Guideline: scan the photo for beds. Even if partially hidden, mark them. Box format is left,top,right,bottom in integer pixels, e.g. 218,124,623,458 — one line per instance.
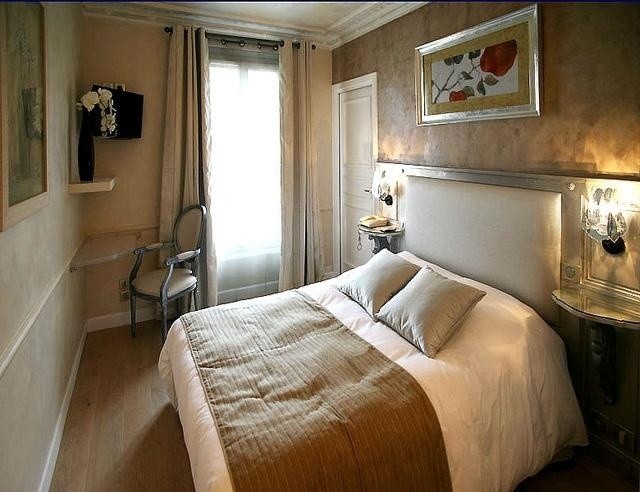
154,179,587,491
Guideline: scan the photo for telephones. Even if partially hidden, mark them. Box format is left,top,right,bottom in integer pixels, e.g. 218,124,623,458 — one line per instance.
359,215,389,227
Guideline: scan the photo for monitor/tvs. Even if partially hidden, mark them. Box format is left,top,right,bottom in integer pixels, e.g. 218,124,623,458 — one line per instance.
90,84,144,140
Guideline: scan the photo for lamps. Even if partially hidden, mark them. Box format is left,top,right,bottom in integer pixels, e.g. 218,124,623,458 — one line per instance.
371,179,393,206
581,205,628,254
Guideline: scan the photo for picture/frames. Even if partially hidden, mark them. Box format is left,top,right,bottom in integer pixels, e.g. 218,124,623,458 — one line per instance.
1,1,53,232
412,3,543,128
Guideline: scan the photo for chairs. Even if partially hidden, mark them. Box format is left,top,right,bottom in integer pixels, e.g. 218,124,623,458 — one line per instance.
128,203,208,347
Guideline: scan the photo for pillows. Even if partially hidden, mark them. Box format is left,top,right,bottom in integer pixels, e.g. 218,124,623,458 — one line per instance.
333,247,488,361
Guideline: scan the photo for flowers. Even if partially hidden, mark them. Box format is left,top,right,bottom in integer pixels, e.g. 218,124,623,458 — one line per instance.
76,88,119,139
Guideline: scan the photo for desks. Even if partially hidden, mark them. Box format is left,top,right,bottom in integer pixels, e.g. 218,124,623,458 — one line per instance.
551,289,640,406
358,225,403,258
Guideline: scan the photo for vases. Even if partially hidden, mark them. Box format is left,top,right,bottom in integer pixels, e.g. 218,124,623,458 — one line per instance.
77,105,95,182
